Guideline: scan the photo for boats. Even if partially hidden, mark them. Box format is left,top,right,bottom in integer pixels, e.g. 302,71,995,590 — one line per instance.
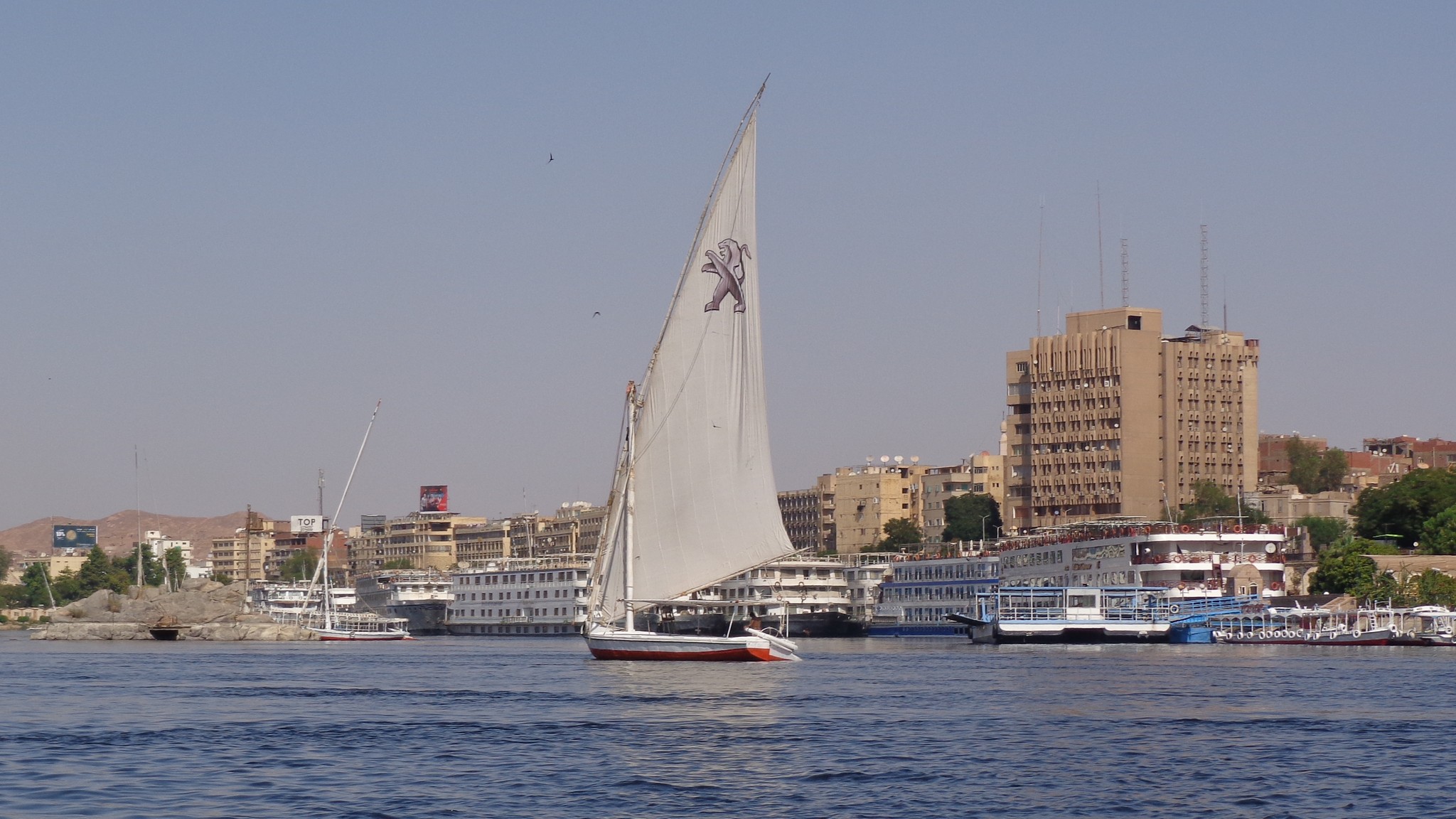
149,626,192,640
653,612,727,637
1301,623,1397,645
1168,613,1218,644
735,611,853,638
940,585,1174,647
1418,623,1456,646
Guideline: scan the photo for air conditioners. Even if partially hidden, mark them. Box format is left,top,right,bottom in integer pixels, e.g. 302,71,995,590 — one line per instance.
1062,448,1067,453
1075,469,1080,474
1193,427,1199,432
1035,490,1115,498
1188,427,1192,431
1091,446,1098,451
1031,381,1110,394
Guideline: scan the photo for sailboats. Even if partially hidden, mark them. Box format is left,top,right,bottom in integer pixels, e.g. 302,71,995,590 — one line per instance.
297,400,413,641
581,70,804,664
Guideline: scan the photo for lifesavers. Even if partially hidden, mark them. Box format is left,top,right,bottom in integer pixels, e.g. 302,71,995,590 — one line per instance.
777,595,782,601
356,569,448,583
1169,604,1180,614
1445,625,1453,634
1215,625,1362,641
775,581,780,587
799,582,804,587
1390,624,1397,633
1281,554,1286,562
893,549,989,562
1234,525,1242,533
1271,582,1279,590
1004,526,1151,551
1181,525,1189,533
1173,555,1181,563
1177,582,1187,589
1154,555,1161,564
801,595,806,601
1249,554,1256,563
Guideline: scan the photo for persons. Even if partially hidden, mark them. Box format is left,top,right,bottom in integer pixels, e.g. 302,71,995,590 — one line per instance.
420,493,442,511
743,612,761,636
